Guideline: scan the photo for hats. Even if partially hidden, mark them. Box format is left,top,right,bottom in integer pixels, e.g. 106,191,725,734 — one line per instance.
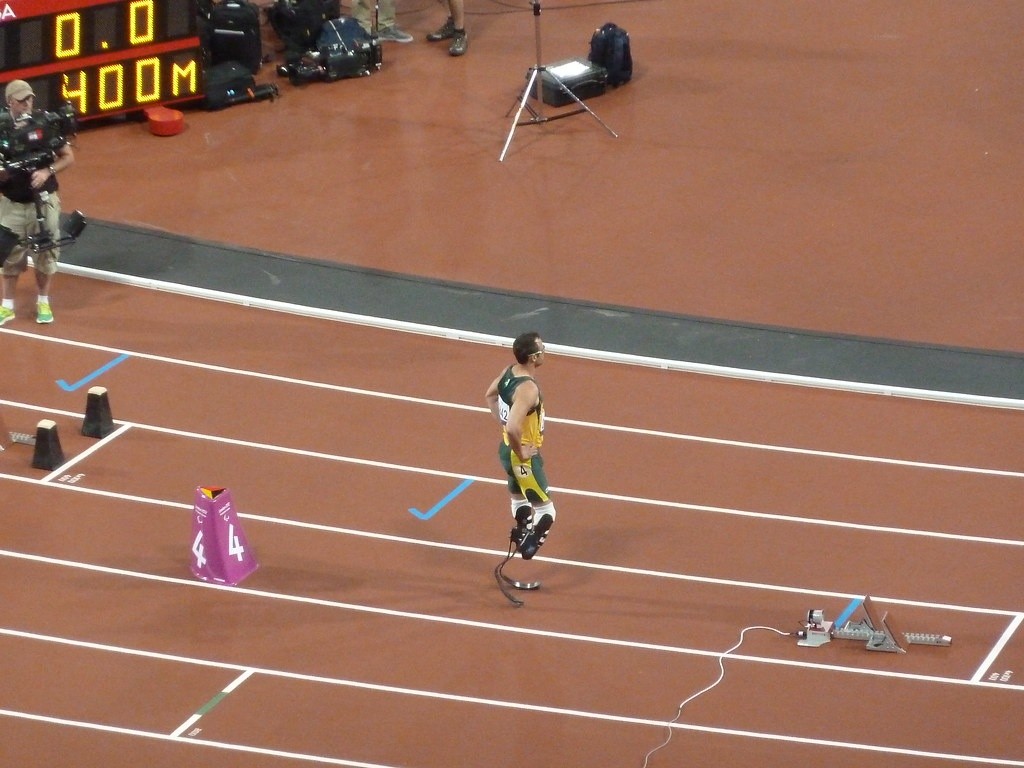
5,79,36,100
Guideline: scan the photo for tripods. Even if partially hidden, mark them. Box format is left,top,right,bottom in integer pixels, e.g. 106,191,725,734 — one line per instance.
498,0,618,162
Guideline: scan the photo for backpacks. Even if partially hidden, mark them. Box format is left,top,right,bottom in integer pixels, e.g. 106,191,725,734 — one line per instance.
317,16,370,52
588,23,632,88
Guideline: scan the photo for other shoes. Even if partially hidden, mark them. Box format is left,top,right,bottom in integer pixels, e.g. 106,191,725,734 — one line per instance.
378,26,413,43
449,33,468,56
426,23,454,41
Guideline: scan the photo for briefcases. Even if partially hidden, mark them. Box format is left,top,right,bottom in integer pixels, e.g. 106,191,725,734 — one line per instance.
209,0,261,77
525,57,608,108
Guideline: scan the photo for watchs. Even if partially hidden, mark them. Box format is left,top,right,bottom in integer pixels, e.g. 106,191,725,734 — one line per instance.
47,164,56,175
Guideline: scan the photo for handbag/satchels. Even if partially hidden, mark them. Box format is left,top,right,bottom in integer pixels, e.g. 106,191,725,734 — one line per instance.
265,0,341,48
205,61,255,105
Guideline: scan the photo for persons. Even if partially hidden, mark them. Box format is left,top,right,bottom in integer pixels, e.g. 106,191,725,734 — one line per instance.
351,0,414,49
426,0,469,56
0,78,76,326
485,331,558,561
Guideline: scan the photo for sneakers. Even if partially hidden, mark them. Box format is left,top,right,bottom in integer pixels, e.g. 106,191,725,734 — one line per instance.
0,306,15,325
36,302,53,323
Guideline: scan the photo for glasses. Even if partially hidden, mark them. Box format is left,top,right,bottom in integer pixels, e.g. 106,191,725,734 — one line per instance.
528,346,545,357
